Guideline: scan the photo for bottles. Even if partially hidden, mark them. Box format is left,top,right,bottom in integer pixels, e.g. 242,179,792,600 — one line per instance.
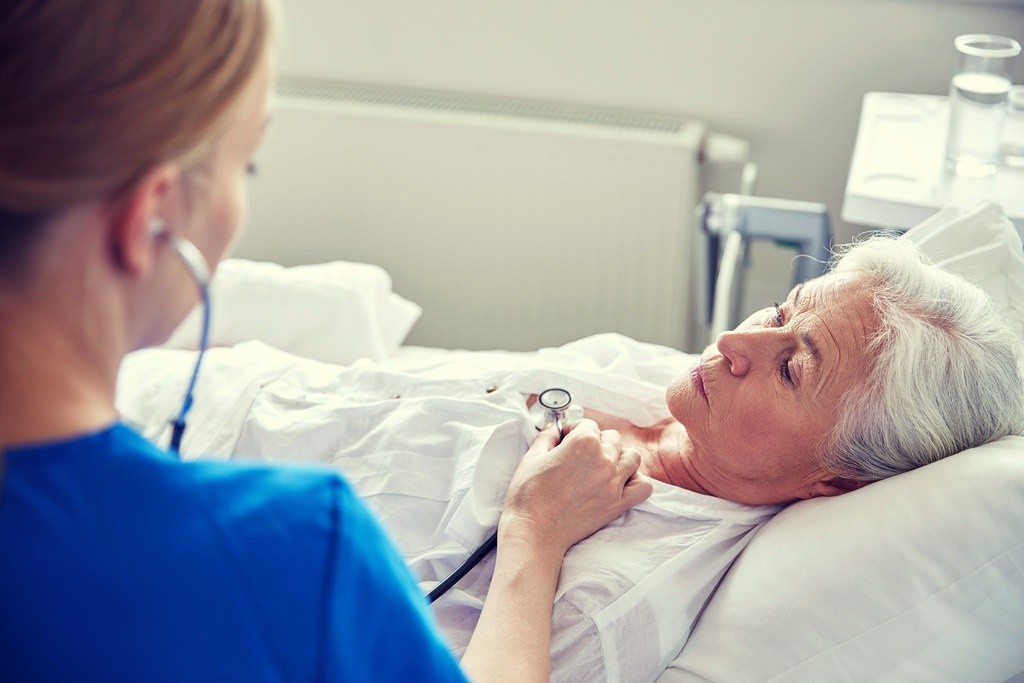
946,74,1010,179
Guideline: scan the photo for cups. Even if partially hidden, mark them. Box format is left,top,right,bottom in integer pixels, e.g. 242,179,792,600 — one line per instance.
955,33,1022,81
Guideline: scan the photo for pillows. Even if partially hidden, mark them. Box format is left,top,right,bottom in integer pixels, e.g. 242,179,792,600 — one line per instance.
654,204,1024,682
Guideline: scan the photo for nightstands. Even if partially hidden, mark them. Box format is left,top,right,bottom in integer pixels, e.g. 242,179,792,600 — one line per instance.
839,89,1024,238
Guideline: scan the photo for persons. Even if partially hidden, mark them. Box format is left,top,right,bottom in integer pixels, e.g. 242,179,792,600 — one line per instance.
114,233,1024,683
0,0,650,683
